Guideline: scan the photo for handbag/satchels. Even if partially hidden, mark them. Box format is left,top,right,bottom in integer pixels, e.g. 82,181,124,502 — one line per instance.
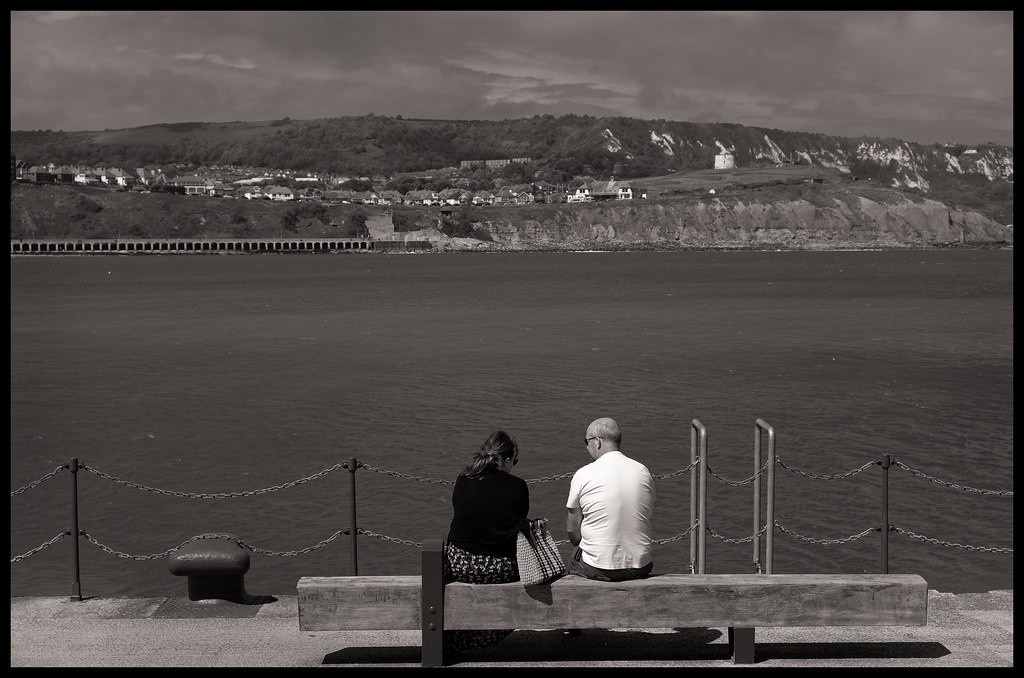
517,516,567,586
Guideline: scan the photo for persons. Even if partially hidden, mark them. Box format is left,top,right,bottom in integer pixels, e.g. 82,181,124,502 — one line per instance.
566,418,657,634
446,430,529,653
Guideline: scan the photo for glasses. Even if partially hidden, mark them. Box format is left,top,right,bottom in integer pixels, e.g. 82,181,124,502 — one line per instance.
508,457,519,466
584,436,604,446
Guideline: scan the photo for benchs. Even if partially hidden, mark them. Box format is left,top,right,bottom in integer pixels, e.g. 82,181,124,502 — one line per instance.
297,574,929,663
